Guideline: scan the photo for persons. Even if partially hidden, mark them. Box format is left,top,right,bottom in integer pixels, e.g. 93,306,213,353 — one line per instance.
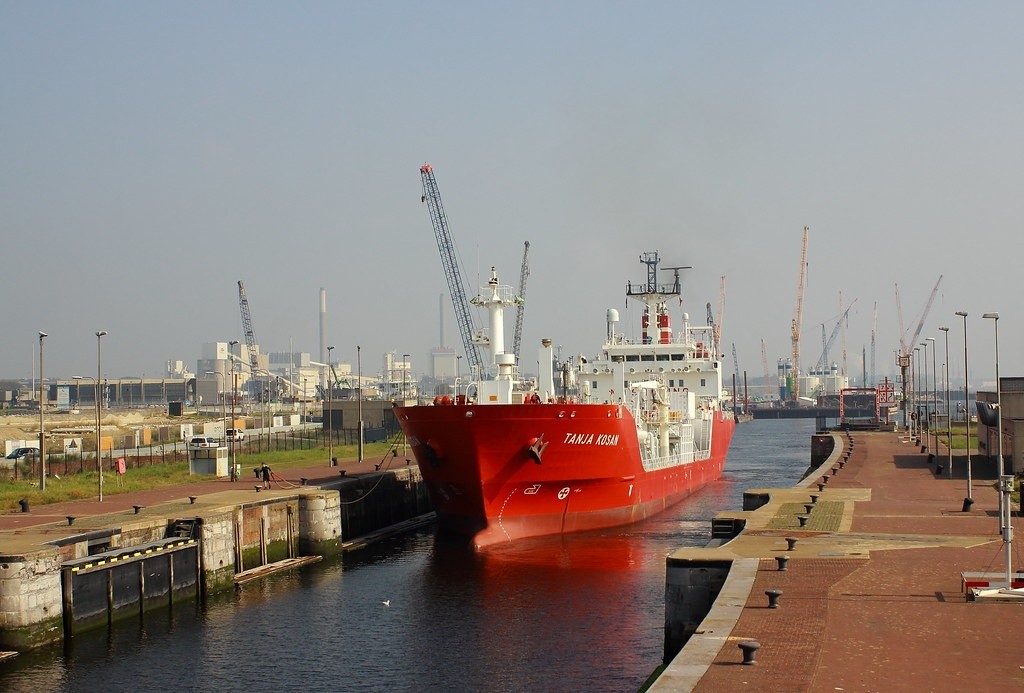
259,462,272,490
390,399,399,408
531,392,543,403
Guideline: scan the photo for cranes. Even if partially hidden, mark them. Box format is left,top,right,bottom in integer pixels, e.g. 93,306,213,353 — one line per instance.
731,343,741,393
511,240,530,366
419,160,486,382
761,338,771,394
713,275,725,353
791,224,809,402
237,280,262,402
816,289,859,378
870,302,877,388
894,273,944,379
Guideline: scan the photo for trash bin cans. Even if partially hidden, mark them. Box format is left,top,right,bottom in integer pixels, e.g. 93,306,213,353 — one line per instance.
1019,481,1024,515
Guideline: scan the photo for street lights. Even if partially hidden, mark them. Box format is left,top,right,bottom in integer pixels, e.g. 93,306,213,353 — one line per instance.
207,340,270,483
909,338,939,466
955,312,972,499
982,312,1004,535
72,331,110,502
939,327,952,478
456,356,463,395
403,354,410,457
357,346,363,463
39,332,46,492
327,346,335,468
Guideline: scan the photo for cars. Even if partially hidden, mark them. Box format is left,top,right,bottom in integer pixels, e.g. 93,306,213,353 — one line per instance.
226,428,244,442
2,447,40,466
190,436,219,448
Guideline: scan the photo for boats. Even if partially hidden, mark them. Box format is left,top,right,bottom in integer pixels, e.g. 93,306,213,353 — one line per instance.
390,250,736,551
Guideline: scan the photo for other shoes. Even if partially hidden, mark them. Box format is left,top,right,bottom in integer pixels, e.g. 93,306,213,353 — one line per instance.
269,485,271,489
264,487,267,490
